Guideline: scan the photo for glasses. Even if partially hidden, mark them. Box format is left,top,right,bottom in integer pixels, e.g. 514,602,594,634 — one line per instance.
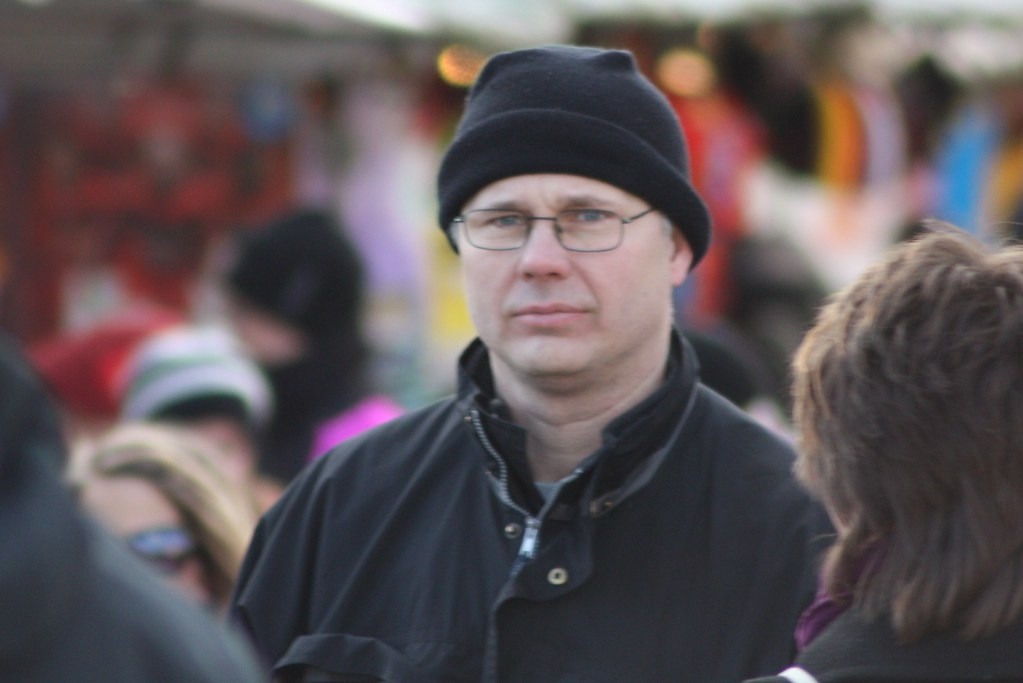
121,527,200,575
449,205,657,253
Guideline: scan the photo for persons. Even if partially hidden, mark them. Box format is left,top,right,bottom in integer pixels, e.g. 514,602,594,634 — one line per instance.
734,216,1023,683
222,206,377,487
223,43,838,683
69,417,255,620
114,319,286,513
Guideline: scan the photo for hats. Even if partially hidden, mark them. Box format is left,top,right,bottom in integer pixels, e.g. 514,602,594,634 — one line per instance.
118,324,274,426
235,212,361,327
437,49,713,274
